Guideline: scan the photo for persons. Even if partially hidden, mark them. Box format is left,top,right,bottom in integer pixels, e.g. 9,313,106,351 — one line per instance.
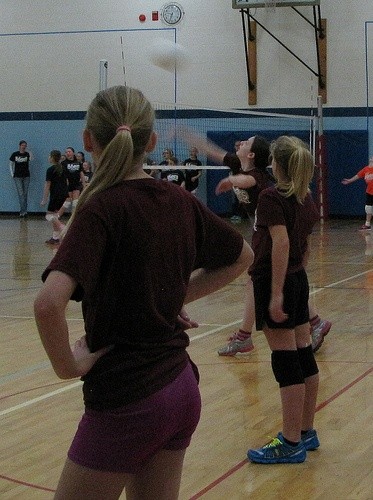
58,145,86,219
167,122,332,357
228,139,244,221
59,151,94,198
34,86,254,500
183,146,202,199
40,149,70,246
9,141,34,221
248,137,320,465
144,148,187,188
342,156,373,232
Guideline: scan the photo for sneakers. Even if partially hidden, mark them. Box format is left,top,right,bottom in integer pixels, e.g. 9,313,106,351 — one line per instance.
302,428,320,451
218,331,254,357
248,432,306,464
310,319,331,351
358,224,371,230
231,215,241,224
45,237,60,245
